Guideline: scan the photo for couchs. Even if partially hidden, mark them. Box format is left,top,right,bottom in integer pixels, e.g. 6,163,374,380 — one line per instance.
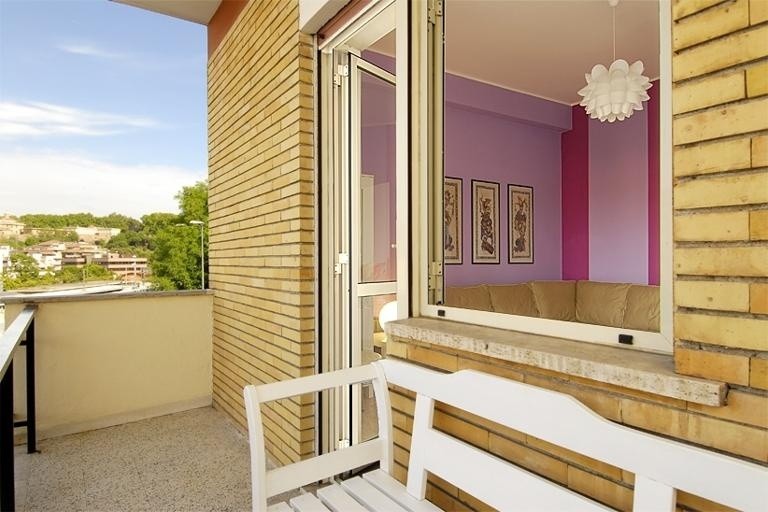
445,279,660,332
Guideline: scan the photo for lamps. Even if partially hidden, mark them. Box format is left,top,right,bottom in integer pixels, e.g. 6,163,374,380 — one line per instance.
577,1,653,123
379,299,398,343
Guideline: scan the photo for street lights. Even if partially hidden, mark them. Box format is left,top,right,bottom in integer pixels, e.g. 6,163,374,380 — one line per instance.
131,255,137,285
189,219,206,290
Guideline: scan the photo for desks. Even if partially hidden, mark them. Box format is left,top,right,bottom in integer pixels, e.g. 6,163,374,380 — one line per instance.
373,330,386,359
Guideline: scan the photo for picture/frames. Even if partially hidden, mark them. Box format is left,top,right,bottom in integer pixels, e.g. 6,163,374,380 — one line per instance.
443,178,463,264
469,178,501,266
506,183,536,263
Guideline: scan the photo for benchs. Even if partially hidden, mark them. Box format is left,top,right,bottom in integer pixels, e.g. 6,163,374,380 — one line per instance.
242,357,768,511
